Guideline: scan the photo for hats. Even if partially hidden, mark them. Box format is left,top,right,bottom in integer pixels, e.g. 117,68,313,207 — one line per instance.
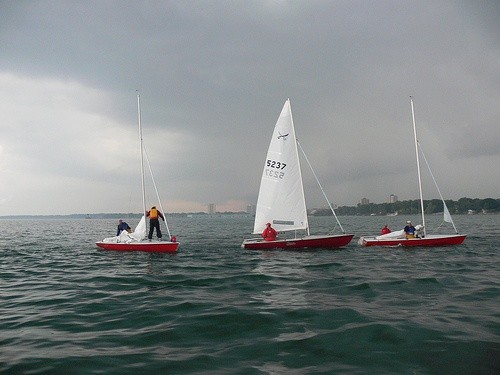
266,223,271,227
407,221,411,223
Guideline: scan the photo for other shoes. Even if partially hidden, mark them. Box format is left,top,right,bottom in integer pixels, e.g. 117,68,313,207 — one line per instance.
159,237,161,239
149,239,151,241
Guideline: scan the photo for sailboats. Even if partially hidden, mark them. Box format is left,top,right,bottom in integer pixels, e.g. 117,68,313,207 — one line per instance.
241,97,355,249
95,94,180,252
358,99,467,247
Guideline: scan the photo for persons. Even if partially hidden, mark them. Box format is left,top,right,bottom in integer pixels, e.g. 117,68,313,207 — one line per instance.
261,223,278,241
404,220,416,235
381,224,391,235
116,219,132,236
146,206,164,239
